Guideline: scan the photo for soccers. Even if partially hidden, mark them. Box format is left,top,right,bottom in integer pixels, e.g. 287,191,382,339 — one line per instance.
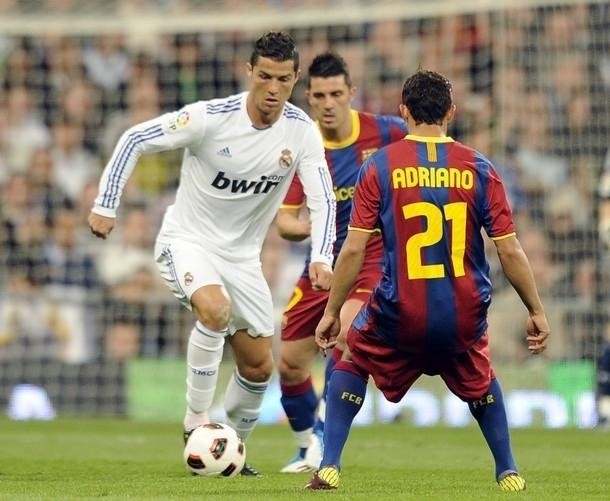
184,423,246,478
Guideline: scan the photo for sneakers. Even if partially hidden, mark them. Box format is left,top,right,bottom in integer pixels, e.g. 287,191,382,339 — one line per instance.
497,469,527,492
183,419,201,444
239,464,259,475
278,426,342,489
